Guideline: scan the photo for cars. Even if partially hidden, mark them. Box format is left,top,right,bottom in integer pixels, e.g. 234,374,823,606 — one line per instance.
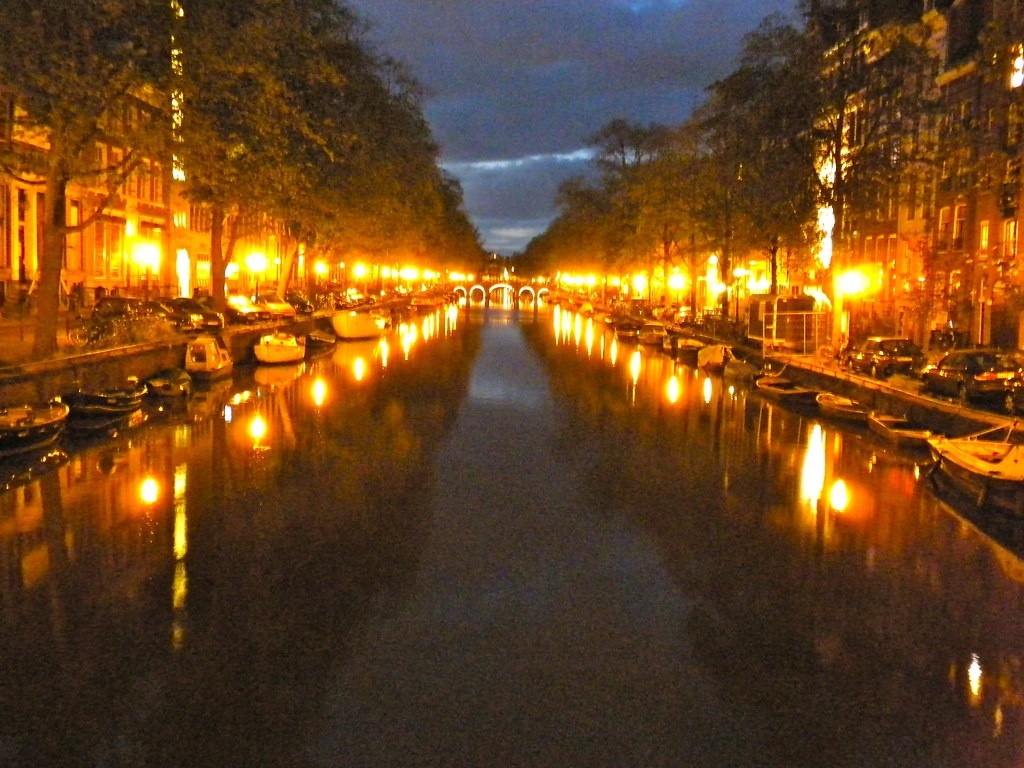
843,335,926,380
920,348,1024,410
88,290,315,344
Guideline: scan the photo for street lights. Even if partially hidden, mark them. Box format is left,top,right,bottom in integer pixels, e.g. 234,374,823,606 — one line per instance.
250,253,266,301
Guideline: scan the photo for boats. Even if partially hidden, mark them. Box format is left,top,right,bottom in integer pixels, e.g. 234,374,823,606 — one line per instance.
142,366,191,398
70,380,148,417
331,313,384,339
865,410,931,448
370,307,394,326
309,329,336,345
406,291,462,308
185,335,234,381
756,375,819,407
815,392,869,424
0,401,70,457
592,311,735,371
254,329,307,364
925,437,1024,519
